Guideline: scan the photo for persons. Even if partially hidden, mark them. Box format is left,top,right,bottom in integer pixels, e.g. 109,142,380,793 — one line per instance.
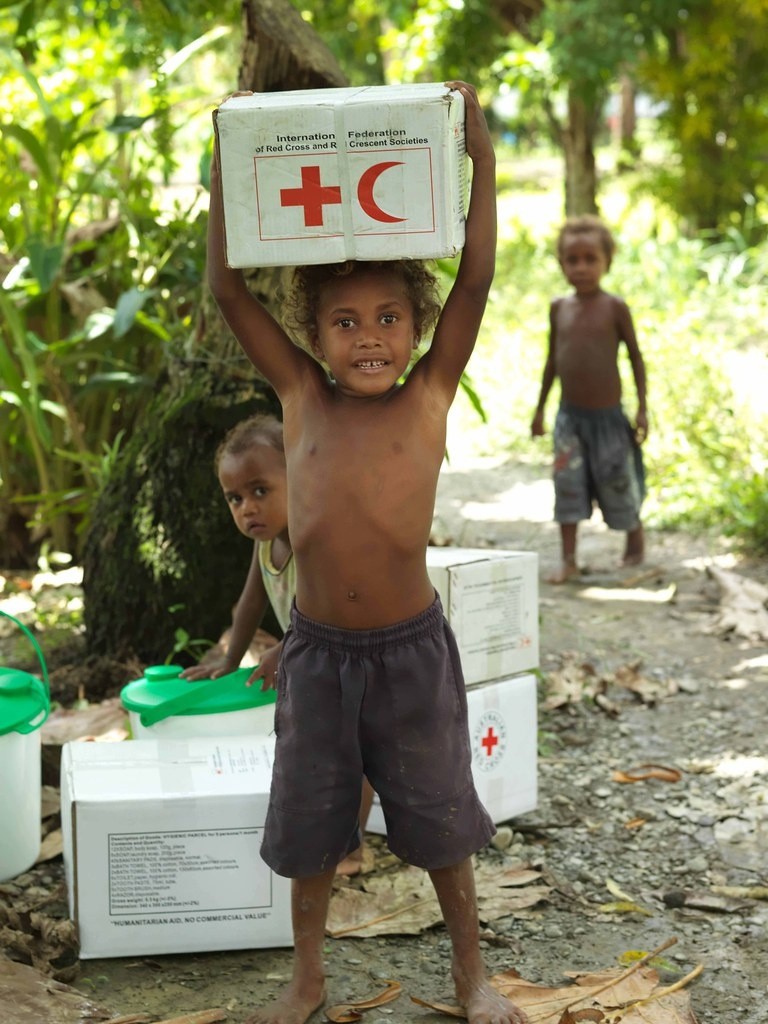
208,82,532,1024
533,216,648,585
179,416,373,877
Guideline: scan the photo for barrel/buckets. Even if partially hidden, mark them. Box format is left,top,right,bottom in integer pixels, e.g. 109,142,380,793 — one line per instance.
118,665,279,739
0,609,50,882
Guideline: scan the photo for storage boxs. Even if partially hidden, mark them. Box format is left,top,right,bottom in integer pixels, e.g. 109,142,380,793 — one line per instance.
364,672,539,835
60,738,294,959
214,79,466,270
425,545,540,684
